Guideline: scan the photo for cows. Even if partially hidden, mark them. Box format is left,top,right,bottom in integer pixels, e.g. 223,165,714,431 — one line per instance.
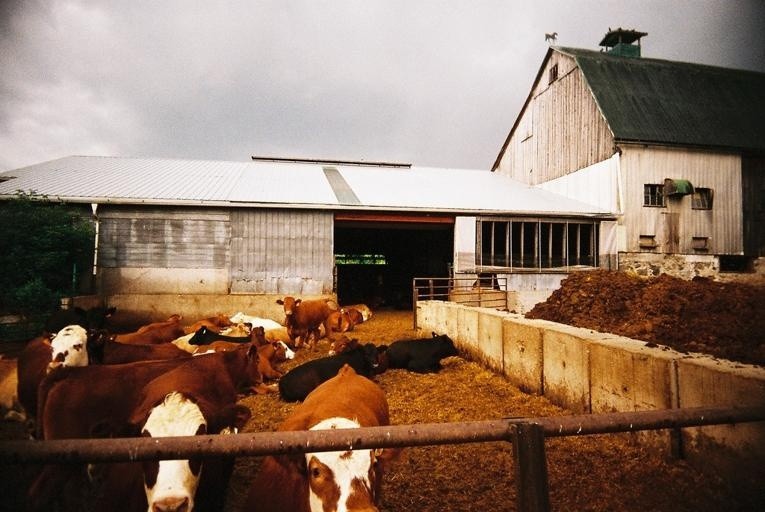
384,332,459,373
243,362,404,512
0,314,296,512
279,342,388,403
277,297,373,352
